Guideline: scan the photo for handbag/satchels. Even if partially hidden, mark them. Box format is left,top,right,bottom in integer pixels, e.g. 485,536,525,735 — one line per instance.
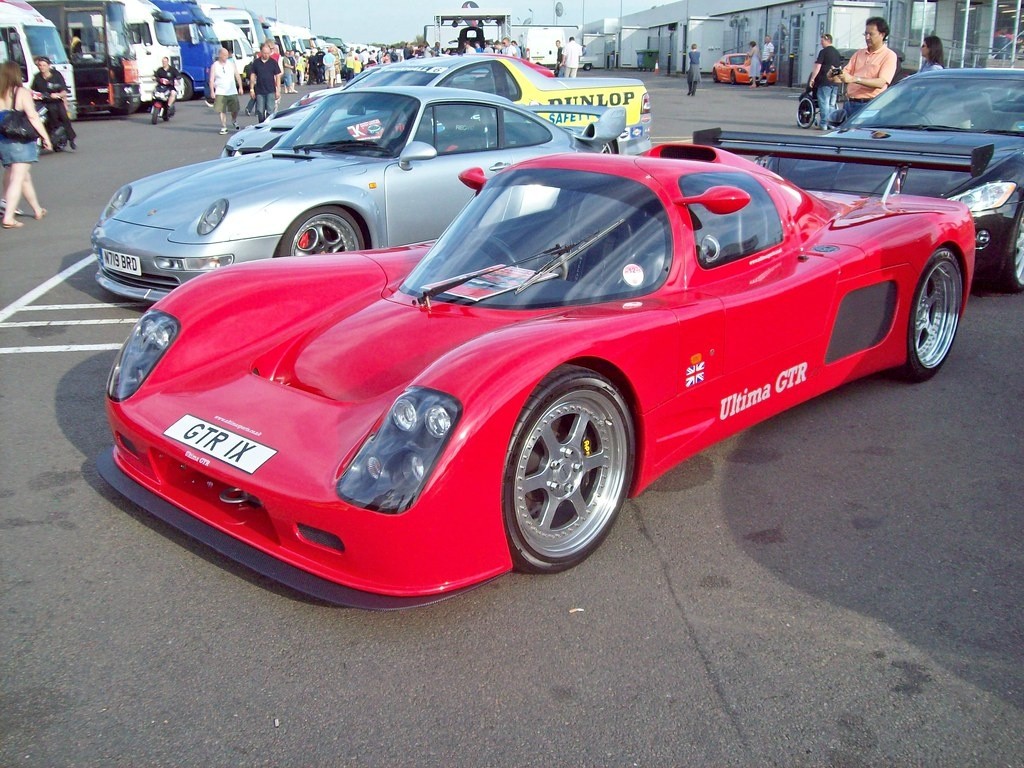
0,86,41,142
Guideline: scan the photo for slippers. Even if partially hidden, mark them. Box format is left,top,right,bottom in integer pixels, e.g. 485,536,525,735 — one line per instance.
3,219,23,229
33,208,46,219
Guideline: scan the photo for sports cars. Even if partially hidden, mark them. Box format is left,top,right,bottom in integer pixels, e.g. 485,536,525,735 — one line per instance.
752,67,1024,293
94,85,628,303
93,128,997,611
713,53,777,85
221,53,651,159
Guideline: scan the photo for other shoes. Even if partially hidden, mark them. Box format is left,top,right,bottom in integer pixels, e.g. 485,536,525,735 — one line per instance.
687,90,693,96
232,122,240,131
1,199,24,215
69,139,77,151
284,90,298,95
815,124,828,132
219,127,228,135
245,108,251,117
800,116,811,124
749,84,756,89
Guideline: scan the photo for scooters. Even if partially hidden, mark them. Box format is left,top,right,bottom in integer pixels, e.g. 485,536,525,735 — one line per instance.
30,83,68,155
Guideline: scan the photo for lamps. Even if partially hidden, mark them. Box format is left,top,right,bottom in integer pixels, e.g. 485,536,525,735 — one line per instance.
437,16,491,28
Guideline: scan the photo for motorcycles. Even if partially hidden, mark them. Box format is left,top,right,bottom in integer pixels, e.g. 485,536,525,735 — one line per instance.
151,70,181,124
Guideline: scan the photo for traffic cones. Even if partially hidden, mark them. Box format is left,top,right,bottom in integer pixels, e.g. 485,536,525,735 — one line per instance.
653,59,660,73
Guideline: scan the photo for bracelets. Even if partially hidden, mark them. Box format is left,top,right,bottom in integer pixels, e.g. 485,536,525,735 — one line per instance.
250,89,254,92
856,77,860,84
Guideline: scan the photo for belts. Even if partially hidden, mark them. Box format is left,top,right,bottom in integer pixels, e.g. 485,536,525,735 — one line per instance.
848,98,869,103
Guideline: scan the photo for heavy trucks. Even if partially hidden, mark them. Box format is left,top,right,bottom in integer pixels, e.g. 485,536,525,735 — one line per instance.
198,5,348,87
0,0,78,127
27,0,142,117
122,0,187,111
149,0,255,101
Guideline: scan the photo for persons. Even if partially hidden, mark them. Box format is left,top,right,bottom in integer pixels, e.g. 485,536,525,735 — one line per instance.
464,38,521,58
282,47,341,93
920,36,944,72
245,40,284,123
758,35,774,86
0,61,52,228
152,57,181,110
206,48,243,134
31,56,77,150
809,34,841,130
561,37,582,78
747,41,760,88
687,44,701,96
345,42,439,81
827,17,897,119
555,40,564,77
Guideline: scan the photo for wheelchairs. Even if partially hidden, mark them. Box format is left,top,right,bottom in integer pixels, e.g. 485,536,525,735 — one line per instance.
797,72,839,129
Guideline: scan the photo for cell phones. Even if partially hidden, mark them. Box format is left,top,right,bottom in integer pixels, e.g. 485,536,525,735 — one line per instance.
832,68,841,76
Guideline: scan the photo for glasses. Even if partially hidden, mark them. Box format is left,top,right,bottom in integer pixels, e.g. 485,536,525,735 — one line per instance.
922,44,929,48
223,67,225,73
865,32,879,36
